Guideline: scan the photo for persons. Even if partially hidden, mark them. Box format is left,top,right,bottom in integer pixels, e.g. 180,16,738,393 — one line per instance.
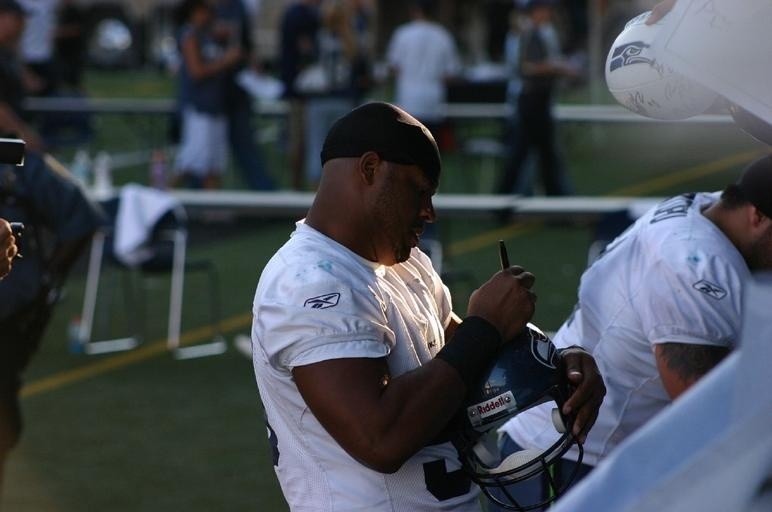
248,99,608,510
489,150,772,511
0,1,772,459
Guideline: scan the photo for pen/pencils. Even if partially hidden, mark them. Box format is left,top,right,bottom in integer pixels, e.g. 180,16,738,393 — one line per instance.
499,240,509,271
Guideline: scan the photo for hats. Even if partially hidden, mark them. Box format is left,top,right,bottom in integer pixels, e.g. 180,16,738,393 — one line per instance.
736,153,772,219
321,101,440,186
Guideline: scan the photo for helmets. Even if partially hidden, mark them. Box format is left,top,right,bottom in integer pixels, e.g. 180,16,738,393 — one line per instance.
604,11,719,121
447,321,584,512
